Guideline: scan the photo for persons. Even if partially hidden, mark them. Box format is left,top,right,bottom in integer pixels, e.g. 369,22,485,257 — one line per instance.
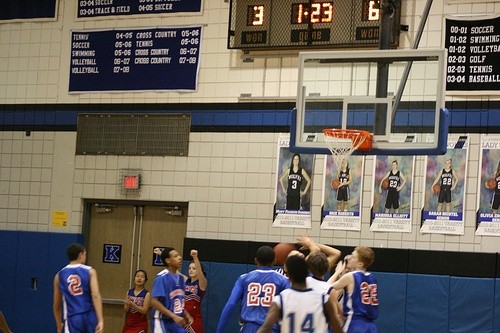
52,242,105,333
431,158,459,212
327,245,379,333
149,247,193,333
330,159,352,211
279,154,311,211
485,161,500,214
255,236,353,333
216,246,291,333
121,269,151,333
154,247,208,333
378,160,407,214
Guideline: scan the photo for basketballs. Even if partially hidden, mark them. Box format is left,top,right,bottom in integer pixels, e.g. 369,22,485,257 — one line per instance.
332,180,340,188
382,180,389,189
274,243,296,266
433,184,440,194
487,179,497,191
